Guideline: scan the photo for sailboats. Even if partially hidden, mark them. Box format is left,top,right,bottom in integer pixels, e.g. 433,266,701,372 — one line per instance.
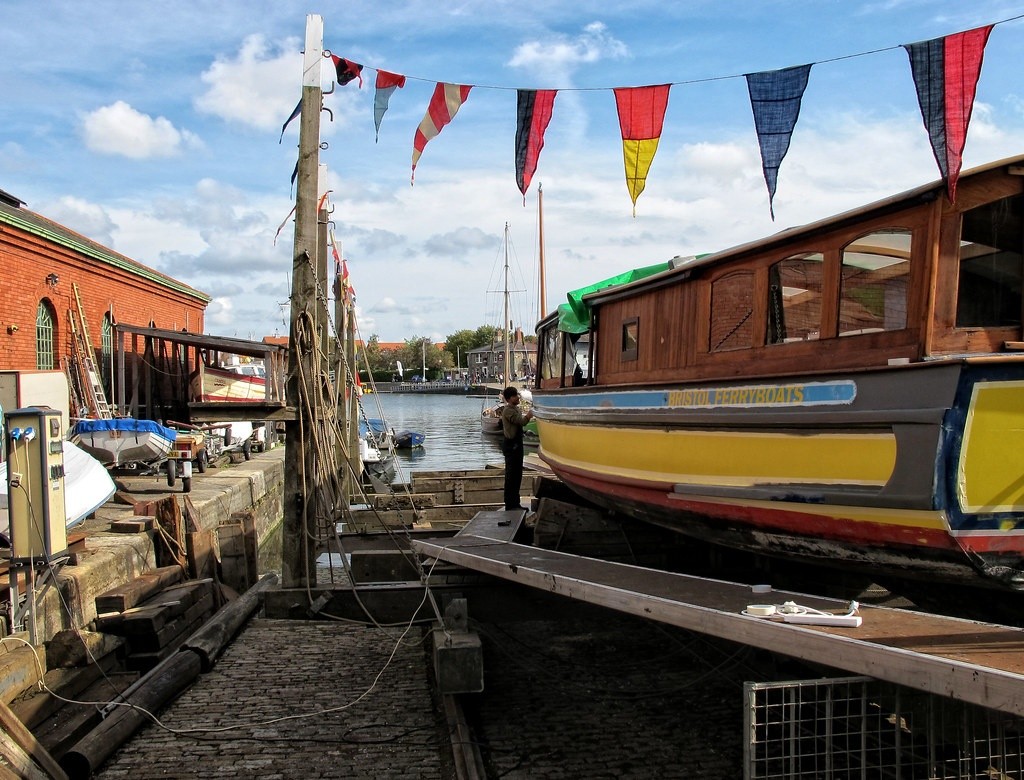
480,180,548,448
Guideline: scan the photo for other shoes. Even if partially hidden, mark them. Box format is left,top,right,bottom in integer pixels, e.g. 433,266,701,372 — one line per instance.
505,504,529,513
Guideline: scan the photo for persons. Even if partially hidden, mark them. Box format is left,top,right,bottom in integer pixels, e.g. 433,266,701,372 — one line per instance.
502,387,534,512
525,373,534,385
495,373,504,383
463,372,480,384
514,370,518,381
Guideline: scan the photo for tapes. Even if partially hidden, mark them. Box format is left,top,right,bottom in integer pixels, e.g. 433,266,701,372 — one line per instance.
746,604,775,615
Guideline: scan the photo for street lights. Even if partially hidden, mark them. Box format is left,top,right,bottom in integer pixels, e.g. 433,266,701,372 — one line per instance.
423,339,429,382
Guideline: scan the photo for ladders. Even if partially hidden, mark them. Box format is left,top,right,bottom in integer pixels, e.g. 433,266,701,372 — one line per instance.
66,282,112,419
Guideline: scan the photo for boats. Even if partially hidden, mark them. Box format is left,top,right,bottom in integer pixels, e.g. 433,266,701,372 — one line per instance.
530,153,1024,593
66,421,177,468
394,431,426,449
0,441,118,549
359,433,394,476
